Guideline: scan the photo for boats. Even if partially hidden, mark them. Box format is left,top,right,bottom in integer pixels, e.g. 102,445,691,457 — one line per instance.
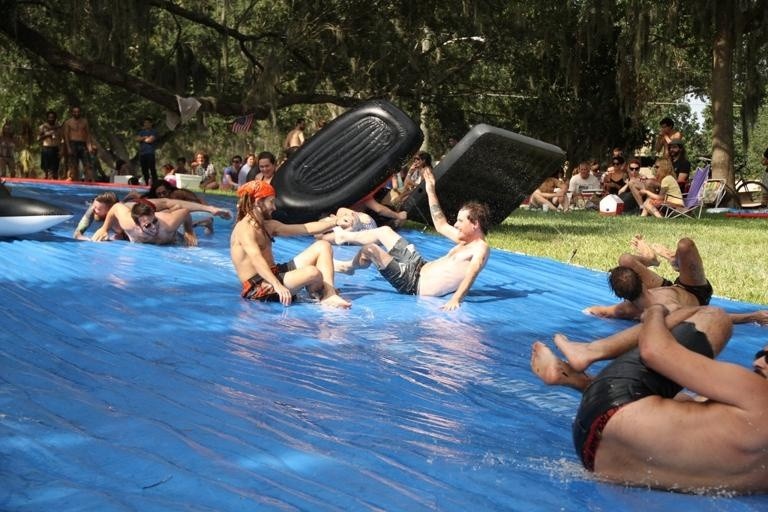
262,98,423,227
0,186,75,239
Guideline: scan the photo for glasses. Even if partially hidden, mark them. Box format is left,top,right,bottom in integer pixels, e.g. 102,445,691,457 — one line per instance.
233,161,239,163
145,219,157,228
629,168,639,170
613,163,619,165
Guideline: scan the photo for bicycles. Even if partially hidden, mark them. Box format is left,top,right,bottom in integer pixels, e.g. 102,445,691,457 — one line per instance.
696,155,768,210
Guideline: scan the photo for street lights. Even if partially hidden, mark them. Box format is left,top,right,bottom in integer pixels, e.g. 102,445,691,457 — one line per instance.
428,36,492,54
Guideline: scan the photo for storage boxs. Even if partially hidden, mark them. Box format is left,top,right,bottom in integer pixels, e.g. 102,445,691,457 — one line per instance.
174,172,202,190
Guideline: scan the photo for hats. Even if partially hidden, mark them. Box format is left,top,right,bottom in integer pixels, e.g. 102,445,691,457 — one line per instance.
669,139,684,147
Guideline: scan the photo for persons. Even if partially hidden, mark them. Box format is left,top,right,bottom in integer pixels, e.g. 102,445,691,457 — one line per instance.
531,308,768,495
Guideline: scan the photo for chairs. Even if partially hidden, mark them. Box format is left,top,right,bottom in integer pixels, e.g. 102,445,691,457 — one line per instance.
701,178,727,208
658,163,711,220
